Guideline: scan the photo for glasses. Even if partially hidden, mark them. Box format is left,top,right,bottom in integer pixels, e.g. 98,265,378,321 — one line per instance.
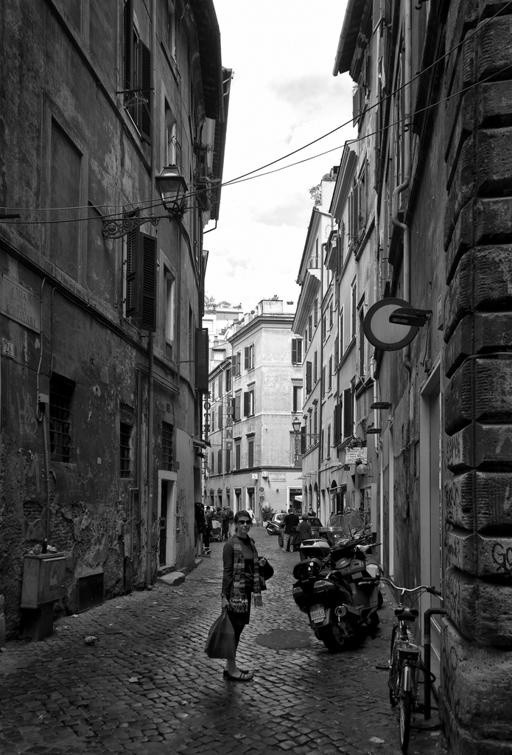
238,520,251,525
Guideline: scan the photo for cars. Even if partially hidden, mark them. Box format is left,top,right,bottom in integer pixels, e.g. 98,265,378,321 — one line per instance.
272,513,324,551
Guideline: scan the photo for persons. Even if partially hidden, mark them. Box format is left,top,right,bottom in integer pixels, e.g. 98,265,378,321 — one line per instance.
281,508,299,553
204,505,230,551
296,514,312,541
221,510,267,682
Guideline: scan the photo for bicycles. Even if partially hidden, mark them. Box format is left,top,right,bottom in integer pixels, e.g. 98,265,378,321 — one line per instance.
362,577,443,755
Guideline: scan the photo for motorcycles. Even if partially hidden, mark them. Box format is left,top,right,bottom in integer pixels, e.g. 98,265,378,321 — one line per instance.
292,512,382,649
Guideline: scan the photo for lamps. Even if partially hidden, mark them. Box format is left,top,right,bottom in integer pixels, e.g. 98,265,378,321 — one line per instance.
100,162,188,241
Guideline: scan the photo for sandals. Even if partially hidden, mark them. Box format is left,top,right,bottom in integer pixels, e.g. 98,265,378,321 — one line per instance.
223,667,254,682
